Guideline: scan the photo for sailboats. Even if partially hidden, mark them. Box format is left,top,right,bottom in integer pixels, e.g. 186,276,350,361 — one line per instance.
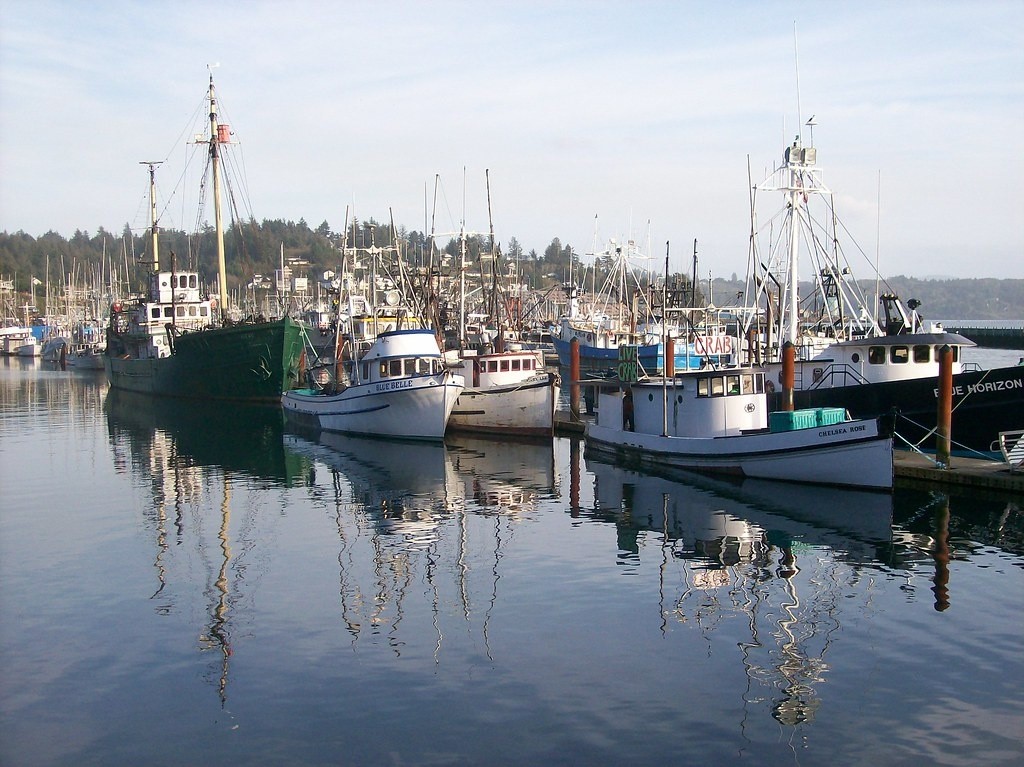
0,21,872,446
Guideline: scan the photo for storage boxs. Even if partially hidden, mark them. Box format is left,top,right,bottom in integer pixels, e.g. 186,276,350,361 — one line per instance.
769,407,846,433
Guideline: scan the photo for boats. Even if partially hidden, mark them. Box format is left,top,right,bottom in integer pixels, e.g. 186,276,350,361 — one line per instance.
700,23,1024,452
576,284,898,490
